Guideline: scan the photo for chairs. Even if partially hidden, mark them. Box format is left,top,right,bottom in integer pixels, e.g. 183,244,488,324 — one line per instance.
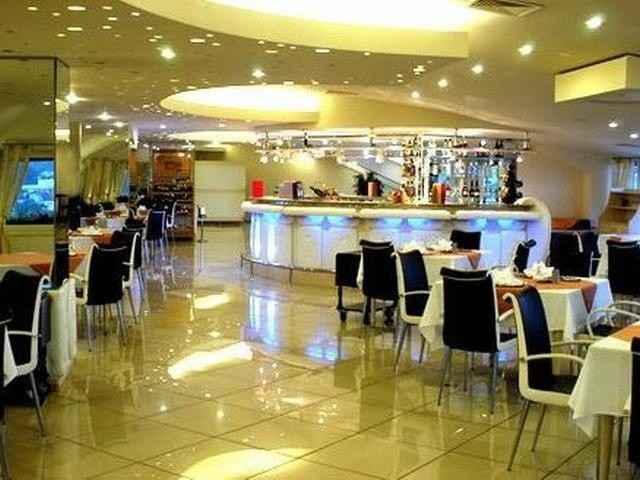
437,268,519,415
337,219,640,369
1,186,205,477
502,285,623,479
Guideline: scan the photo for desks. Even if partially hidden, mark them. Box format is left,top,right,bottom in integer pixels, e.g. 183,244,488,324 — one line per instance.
569,321,640,479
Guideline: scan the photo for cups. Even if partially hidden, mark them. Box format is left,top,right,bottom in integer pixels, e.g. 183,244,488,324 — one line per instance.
431,183,445,203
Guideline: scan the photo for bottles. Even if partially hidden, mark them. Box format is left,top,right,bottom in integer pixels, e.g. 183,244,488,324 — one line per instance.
462,179,481,206
310,185,326,199
262,127,379,149
400,138,424,198
429,128,531,165
